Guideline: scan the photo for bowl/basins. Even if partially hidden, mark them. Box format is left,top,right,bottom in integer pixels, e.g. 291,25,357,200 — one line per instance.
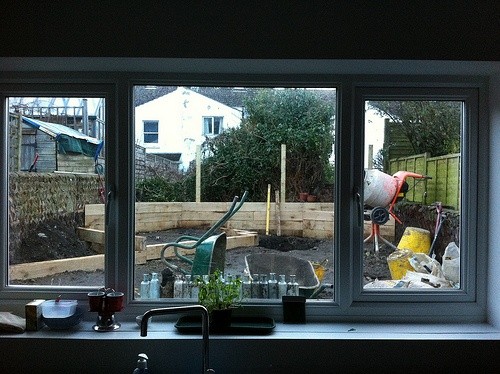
135,315,152,327
40,299,83,330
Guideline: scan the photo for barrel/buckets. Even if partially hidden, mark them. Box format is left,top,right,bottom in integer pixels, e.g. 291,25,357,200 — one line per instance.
395,227,431,256
312,264,324,280
386,248,415,279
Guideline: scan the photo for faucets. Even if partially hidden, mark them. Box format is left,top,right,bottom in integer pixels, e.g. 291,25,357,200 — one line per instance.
141,305,216,374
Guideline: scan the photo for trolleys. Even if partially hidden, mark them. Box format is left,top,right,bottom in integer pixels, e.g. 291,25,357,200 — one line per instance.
161,190,250,283
244,253,333,299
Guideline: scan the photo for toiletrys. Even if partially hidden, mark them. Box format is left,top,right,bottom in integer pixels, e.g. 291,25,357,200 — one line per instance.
134,353,148,374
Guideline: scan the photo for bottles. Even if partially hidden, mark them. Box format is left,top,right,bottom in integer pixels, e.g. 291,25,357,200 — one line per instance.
173,272,300,301
140,272,161,299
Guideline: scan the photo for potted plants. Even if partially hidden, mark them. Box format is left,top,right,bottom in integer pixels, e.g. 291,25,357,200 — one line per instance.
193,267,243,328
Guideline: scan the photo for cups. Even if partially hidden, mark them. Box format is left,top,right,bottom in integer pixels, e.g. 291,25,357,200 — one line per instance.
88,291,104,311
107,291,124,312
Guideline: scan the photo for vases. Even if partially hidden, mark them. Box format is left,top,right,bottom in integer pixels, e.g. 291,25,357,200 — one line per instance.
307,194,317,202
299,193,309,201
